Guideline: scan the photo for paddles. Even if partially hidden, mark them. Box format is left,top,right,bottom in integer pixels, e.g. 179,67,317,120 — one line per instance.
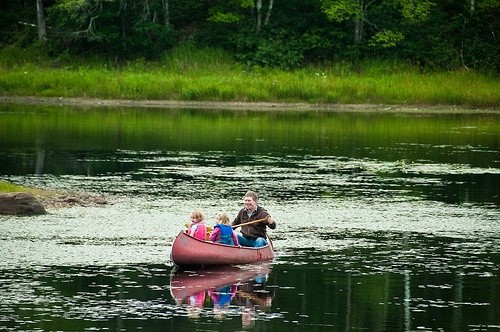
206,214,269,241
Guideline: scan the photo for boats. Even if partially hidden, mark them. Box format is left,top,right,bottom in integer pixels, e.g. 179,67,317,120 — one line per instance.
170,264,269,301
171,230,273,265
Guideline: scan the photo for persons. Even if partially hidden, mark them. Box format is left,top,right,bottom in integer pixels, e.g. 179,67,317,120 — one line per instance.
230,191,276,248
208,212,242,248
186,272,273,329
188,211,207,241
